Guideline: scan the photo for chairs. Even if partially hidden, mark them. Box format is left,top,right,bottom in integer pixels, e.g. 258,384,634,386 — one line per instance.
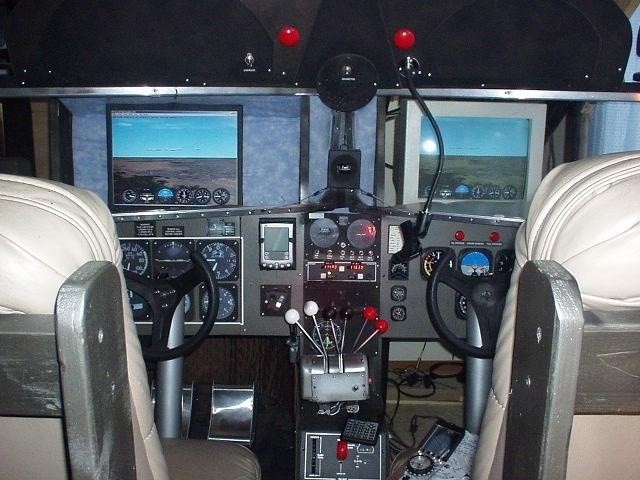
471,147,640,480
1,173,261,479
